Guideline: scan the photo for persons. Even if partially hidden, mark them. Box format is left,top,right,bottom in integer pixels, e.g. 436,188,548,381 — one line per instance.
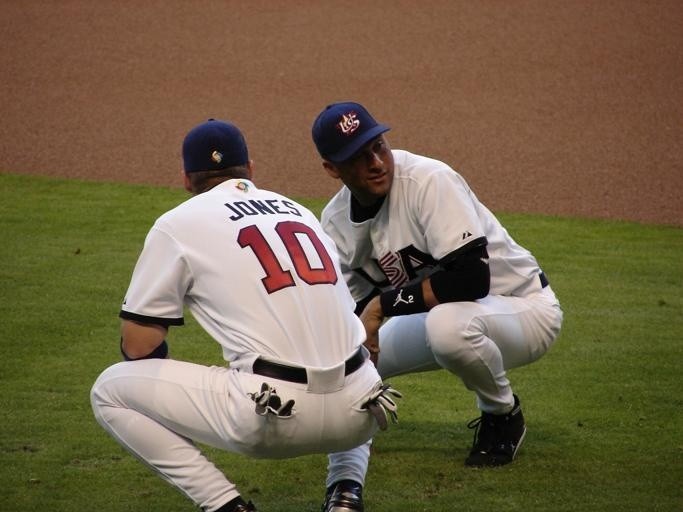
300,101,566,463
88,120,388,511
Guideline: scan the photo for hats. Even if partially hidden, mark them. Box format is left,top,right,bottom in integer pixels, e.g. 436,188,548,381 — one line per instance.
182,118,248,173
312,102,391,162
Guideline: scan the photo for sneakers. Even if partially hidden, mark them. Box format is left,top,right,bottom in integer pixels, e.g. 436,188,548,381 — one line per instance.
465,393,526,468
320,482,363,512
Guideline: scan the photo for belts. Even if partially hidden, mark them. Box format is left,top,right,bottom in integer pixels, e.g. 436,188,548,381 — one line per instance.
254,345,369,383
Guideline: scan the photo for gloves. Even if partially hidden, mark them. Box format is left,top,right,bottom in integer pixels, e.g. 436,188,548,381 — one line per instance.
248,383,295,417
361,383,403,430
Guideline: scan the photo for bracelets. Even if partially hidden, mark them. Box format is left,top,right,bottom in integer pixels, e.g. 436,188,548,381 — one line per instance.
380,281,431,315
119,331,168,360
